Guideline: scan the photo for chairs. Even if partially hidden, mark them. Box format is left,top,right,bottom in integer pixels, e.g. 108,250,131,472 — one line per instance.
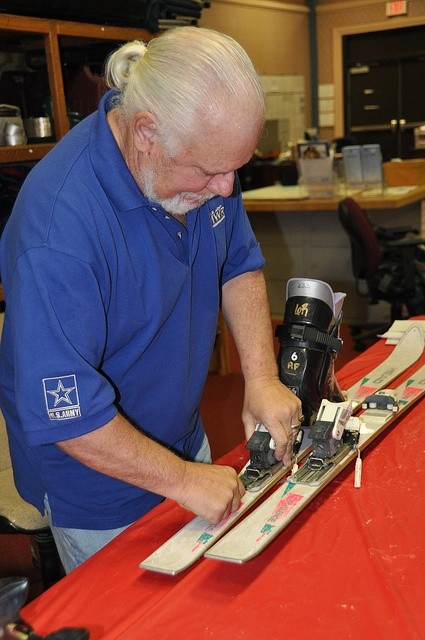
334,197,424,350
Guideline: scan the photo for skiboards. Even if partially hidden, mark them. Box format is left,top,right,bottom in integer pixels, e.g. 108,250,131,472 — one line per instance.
138,323,425,575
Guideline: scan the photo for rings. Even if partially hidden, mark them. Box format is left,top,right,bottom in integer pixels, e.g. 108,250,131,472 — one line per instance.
298,415,305,422
290,423,300,430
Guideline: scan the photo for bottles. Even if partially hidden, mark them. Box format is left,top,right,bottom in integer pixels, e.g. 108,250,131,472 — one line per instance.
33,107,54,143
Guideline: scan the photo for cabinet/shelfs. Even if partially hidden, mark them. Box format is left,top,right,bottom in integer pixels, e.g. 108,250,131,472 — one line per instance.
0,14,156,231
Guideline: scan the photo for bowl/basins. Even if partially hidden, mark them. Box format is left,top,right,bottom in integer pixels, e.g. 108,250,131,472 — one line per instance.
3,121,23,134
4,135,26,145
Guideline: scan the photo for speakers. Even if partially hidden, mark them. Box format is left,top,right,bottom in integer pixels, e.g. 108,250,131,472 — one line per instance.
362,143,383,193
342,146,362,190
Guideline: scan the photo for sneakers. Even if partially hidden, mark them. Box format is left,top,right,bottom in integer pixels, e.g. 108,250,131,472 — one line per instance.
274,278,347,426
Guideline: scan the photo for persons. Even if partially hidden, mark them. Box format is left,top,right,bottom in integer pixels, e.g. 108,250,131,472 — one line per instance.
1,25,305,576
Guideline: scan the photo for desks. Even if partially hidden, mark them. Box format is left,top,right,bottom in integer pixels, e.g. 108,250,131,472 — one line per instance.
240,185,425,324
20,314,425,639
